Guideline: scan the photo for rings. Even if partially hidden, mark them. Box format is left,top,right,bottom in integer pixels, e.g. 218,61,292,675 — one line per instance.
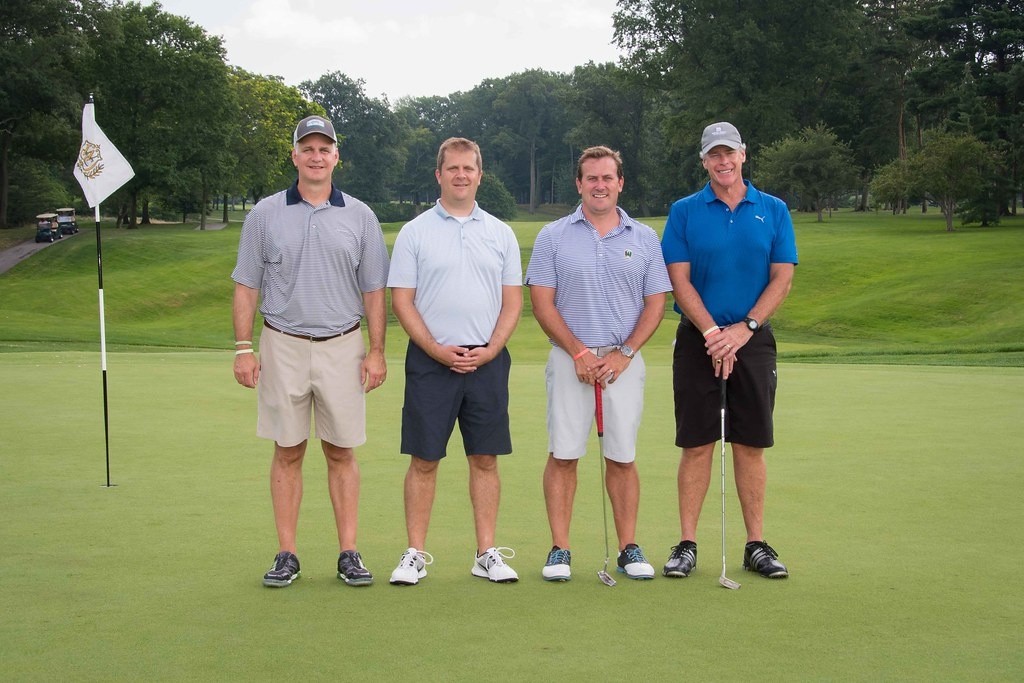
725,344,731,351
716,359,721,363
380,381,383,383
610,369,613,373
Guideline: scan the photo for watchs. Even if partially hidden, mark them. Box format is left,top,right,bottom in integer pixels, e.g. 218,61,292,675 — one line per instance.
743,317,760,336
620,343,635,359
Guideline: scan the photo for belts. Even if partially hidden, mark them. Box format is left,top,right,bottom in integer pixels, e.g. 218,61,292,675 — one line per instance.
261,319,360,342
587,347,617,357
679,315,769,335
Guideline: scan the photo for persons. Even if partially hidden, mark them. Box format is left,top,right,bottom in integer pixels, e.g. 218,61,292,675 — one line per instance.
525,145,676,581
230,113,388,587
663,121,799,578
386,138,523,584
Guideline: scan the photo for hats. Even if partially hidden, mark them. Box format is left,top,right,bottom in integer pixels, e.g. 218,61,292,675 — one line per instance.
294,116,337,142
701,121,742,155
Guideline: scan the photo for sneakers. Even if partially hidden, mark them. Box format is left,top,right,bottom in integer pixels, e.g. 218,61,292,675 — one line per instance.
262,551,301,587
337,553,374,585
541,545,571,582
661,540,698,575
743,540,788,578
389,548,433,584
616,543,654,579
471,546,519,582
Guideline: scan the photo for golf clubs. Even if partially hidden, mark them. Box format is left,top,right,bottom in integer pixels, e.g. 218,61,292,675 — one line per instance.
718,369,741,590
594,374,617,586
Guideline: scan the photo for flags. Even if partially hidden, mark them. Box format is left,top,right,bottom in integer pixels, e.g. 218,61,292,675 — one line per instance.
73,102,134,209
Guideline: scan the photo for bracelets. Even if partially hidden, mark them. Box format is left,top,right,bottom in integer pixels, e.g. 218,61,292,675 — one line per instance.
235,341,253,345
702,326,719,337
235,348,253,355
573,348,590,361
